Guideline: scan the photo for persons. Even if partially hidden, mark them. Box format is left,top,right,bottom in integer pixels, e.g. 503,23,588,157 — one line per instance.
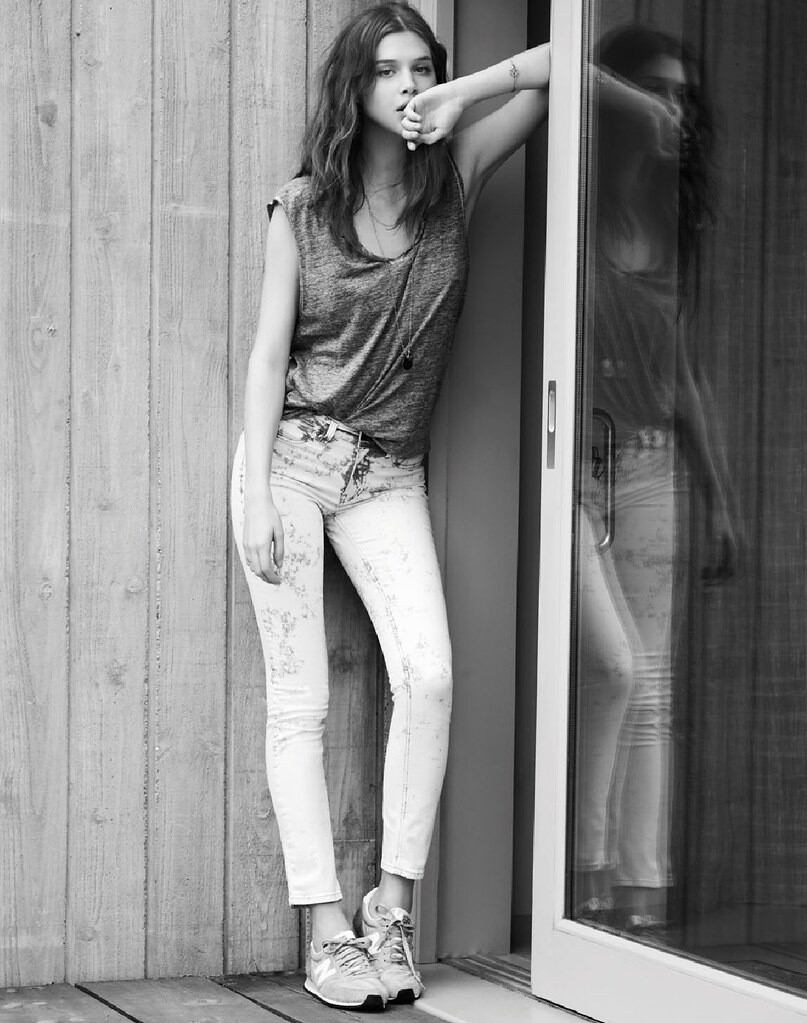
230,0,551,1013
574,18,742,944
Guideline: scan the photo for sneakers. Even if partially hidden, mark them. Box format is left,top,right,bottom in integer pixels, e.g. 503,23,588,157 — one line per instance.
577,898,615,927
354,888,425,1004
304,931,389,1012
624,915,666,945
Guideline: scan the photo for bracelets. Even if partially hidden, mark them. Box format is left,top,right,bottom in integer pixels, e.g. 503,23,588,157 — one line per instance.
596,68,607,87
508,56,520,93
